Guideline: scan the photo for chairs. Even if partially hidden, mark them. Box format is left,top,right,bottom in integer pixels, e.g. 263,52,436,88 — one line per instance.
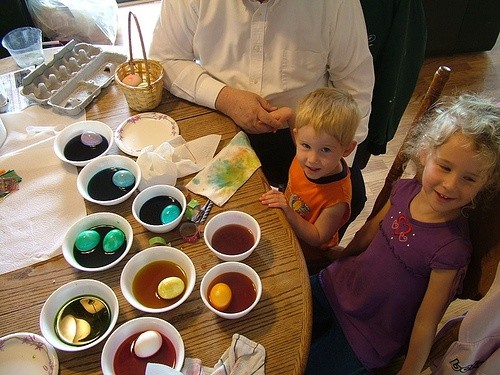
356,66,500,375
354,0,428,168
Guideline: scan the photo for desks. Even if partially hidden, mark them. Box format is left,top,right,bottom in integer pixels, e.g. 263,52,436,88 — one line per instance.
0,45,313,375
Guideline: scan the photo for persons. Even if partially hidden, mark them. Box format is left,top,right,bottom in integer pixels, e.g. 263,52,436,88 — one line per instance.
258,87,360,267
148,0,375,244
300,97,500,375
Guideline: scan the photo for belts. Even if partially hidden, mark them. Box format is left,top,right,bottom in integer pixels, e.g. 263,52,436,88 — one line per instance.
265,127,291,135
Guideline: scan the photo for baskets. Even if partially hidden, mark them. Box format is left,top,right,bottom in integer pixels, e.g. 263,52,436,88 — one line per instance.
115,11,164,112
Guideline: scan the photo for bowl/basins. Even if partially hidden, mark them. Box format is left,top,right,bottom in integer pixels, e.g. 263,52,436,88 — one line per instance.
200,261,264,319
61,212,134,272
1,27,45,69
39,279,120,352
132,184,187,234
119,246,196,314
77,155,142,206
100,317,185,375
53,120,114,166
204,210,263,261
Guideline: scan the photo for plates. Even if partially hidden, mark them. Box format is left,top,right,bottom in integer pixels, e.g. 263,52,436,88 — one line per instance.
0,332,59,375
115,112,181,156
